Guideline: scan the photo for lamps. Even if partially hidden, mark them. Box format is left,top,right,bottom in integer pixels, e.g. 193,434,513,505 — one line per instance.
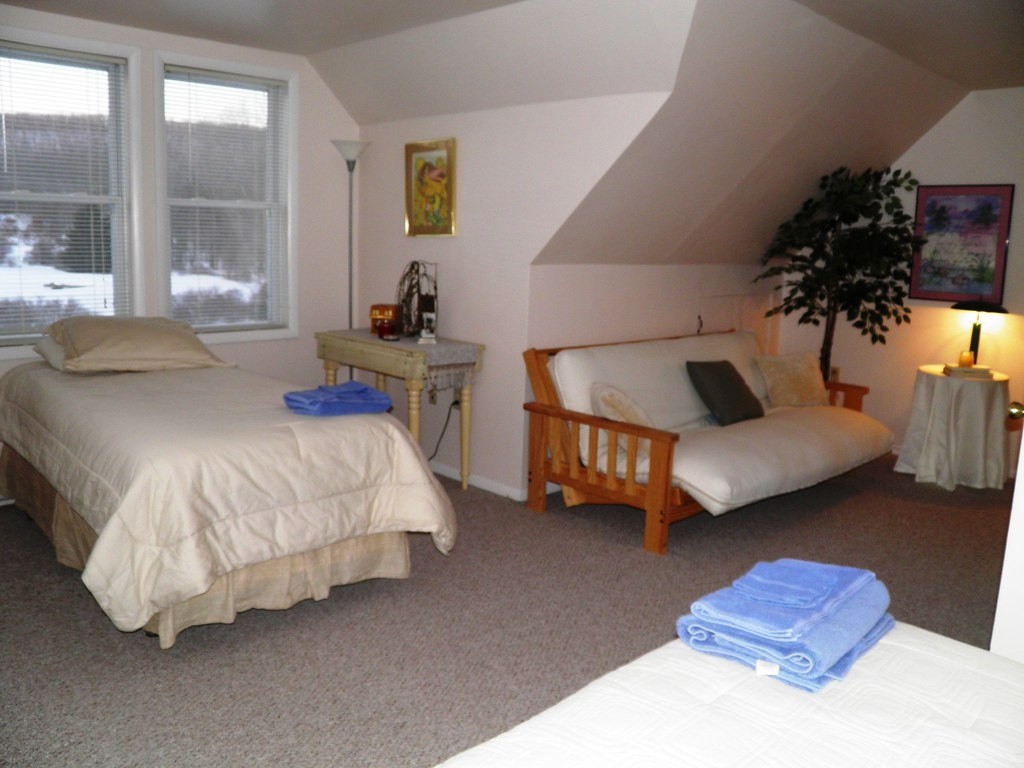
327,138,369,381
945,302,1010,368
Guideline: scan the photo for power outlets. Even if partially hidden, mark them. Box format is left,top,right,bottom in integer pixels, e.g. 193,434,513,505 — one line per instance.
452,388,461,410
428,390,437,405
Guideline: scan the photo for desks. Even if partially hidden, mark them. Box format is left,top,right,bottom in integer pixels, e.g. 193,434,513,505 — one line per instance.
891,363,1011,490
315,329,487,494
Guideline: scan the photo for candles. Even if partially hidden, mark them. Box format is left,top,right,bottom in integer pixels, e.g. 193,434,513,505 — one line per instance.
959,351,974,368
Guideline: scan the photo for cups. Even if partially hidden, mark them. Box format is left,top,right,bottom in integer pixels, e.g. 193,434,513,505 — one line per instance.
959,352,975,369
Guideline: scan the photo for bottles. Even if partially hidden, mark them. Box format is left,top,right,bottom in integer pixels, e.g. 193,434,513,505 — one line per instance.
379,320,393,338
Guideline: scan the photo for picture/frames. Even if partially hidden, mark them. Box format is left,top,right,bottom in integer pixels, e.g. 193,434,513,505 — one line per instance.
403,137,455,239
906,182,1015,306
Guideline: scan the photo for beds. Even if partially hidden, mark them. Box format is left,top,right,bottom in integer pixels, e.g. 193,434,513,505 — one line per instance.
439,620,1024,768
0,360,460,653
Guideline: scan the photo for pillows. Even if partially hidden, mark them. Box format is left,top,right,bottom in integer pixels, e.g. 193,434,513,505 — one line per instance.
686,356,767,429
44,310,244,376
35,338,67,372
753,349,830,409
589,382,655,458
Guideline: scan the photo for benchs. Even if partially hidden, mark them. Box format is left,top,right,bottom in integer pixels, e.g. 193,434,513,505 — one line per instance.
519,328,896,557
